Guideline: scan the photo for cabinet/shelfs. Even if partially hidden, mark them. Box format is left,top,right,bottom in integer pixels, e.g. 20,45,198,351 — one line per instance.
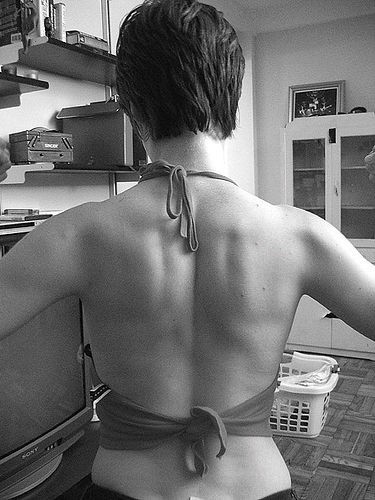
282,112,375,361
0,0,149,257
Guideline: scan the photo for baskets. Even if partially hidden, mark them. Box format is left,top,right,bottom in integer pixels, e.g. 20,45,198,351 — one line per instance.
269,351,341,440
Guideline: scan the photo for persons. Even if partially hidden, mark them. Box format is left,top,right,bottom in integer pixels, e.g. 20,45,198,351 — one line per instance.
0,0,374,500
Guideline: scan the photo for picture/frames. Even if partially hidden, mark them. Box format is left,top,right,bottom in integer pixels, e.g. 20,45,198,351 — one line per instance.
286,79,345,122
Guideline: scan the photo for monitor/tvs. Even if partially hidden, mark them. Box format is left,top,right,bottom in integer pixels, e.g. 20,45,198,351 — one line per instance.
0,231,94,500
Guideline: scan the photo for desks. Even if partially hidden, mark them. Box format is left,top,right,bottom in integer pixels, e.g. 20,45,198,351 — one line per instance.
12,421,103,500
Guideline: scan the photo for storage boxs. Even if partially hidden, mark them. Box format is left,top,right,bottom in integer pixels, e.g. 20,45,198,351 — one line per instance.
269,351,341,439
